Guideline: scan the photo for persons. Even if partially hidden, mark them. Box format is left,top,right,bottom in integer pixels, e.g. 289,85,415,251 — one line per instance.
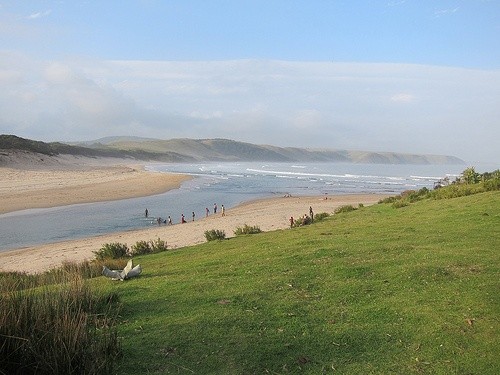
181,214,186,224
156,218,166,224
168,216,172,225
221,205,225,217
145,209,148,216
299,206,313,227
214,203,217,213
289,217,296,227
192,211,196,221
206,208,209,217
